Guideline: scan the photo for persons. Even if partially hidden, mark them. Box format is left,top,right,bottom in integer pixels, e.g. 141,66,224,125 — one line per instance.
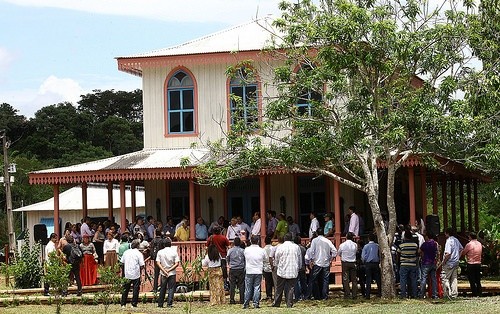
205,225,230,291
130,214,190,263
348,226,482,300
79,234,99,286
195,212,261,246
57,228,76,255
117,235,130,279
322,206,366,238
267,210,300,241
61,235,83,296
226,237,246,304
308,211,320,237
156,238,181,308
243,228,338,309
119,240,145,307
201,244,226,305
103,231,120,268
65,216,135,266
147,230,166,292
43,233,70,295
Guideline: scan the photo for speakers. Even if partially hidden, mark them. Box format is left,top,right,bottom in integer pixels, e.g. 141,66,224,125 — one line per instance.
34,224,47,245
425,214,440,236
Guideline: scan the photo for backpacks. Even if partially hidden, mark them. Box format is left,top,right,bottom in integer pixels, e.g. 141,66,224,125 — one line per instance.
69,244,83,265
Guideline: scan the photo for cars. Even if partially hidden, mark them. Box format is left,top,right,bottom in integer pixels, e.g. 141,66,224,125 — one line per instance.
437,232,492,276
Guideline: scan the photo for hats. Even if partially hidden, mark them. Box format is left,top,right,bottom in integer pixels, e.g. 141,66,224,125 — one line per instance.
323,213,331,217
